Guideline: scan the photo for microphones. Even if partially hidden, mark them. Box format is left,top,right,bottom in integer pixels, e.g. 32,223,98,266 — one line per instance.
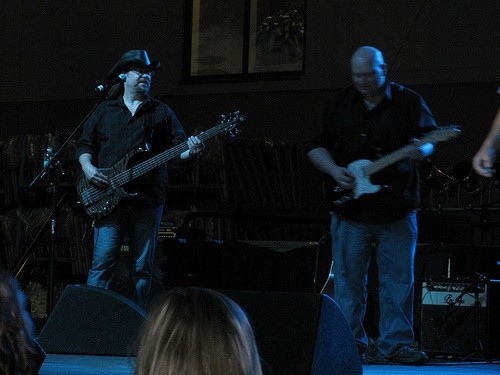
95,74,127,93
447,300,455,308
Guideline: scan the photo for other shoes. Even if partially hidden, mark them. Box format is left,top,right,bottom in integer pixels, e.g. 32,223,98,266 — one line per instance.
388,347,428,364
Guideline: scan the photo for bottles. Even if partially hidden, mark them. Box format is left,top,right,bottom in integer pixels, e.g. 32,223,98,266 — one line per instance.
43,145,61,168
31,282,47,318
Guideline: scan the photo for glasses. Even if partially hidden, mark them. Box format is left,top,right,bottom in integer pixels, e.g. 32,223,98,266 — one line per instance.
126,68,154,77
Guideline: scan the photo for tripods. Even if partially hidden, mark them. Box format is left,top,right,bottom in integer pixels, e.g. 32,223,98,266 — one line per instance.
450,276,493,363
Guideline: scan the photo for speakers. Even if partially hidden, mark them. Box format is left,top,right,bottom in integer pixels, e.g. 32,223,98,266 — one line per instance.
36,282,149,357
419,278,500,362
117,237,226,303
217,289,363,375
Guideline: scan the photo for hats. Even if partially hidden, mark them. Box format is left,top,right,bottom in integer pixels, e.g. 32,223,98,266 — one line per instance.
110,50,160,77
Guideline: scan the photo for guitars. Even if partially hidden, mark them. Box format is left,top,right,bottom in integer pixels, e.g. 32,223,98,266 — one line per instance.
323,124,461,206
76,110,247,219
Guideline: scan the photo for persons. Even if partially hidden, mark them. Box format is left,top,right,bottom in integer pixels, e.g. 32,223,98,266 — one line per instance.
470,88,500,178
77,49,206,296
136,286,263,375
302,45,438,366
0,271,39,375
